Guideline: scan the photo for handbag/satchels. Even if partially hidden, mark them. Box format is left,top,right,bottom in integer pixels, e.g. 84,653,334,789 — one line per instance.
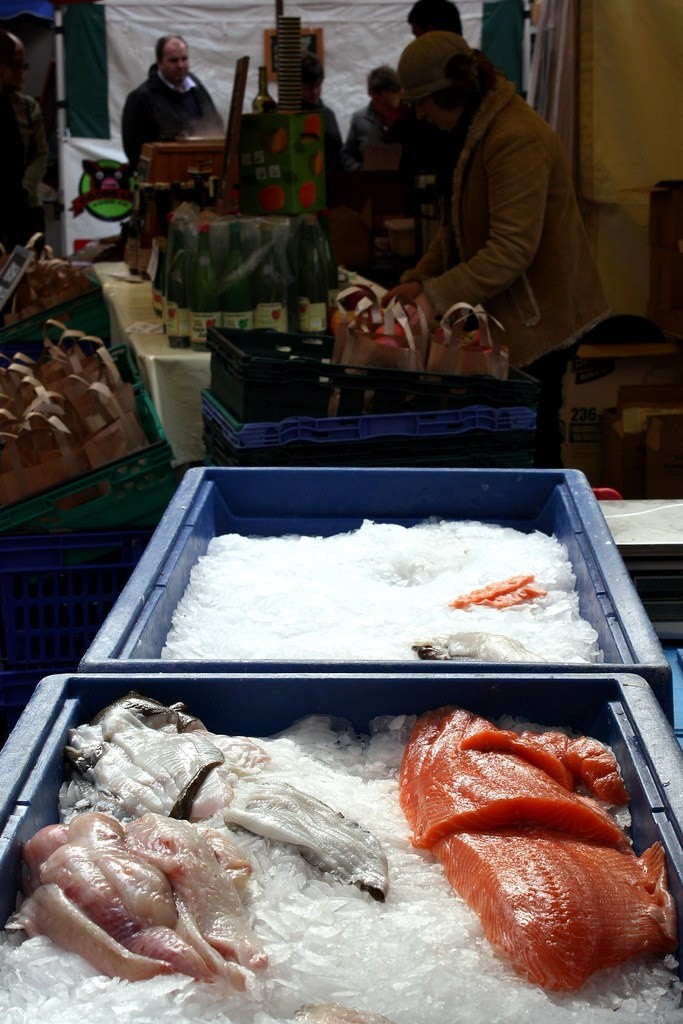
334,284,512,377
1,232,98,324
1,319,156,518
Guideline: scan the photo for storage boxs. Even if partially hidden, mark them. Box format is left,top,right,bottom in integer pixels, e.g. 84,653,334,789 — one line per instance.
199,332,562,520
0,348,183,526
1,281,108,356
642,415,679,492
600,386,680,500
76,467,673,728
0,531,167,734
0,674,682,1023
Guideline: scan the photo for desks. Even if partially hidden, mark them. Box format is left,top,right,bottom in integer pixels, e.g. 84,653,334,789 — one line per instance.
91,258,678,613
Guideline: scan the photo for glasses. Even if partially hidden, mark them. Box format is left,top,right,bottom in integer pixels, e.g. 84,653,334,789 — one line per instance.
4,59,30,73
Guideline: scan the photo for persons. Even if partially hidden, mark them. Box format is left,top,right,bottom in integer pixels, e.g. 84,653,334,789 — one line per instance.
121,36,225,172
298,52,344,208
1,32,51,260
0,28,27,262
379,29,615,470
341,66,407,214
398,0,465,221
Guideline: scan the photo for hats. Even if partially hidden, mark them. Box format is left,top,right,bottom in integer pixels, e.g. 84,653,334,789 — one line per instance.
399,31,477,101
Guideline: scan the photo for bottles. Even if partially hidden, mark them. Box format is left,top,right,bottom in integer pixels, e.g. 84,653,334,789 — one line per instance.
252,66,272,112
124,173,337,353
